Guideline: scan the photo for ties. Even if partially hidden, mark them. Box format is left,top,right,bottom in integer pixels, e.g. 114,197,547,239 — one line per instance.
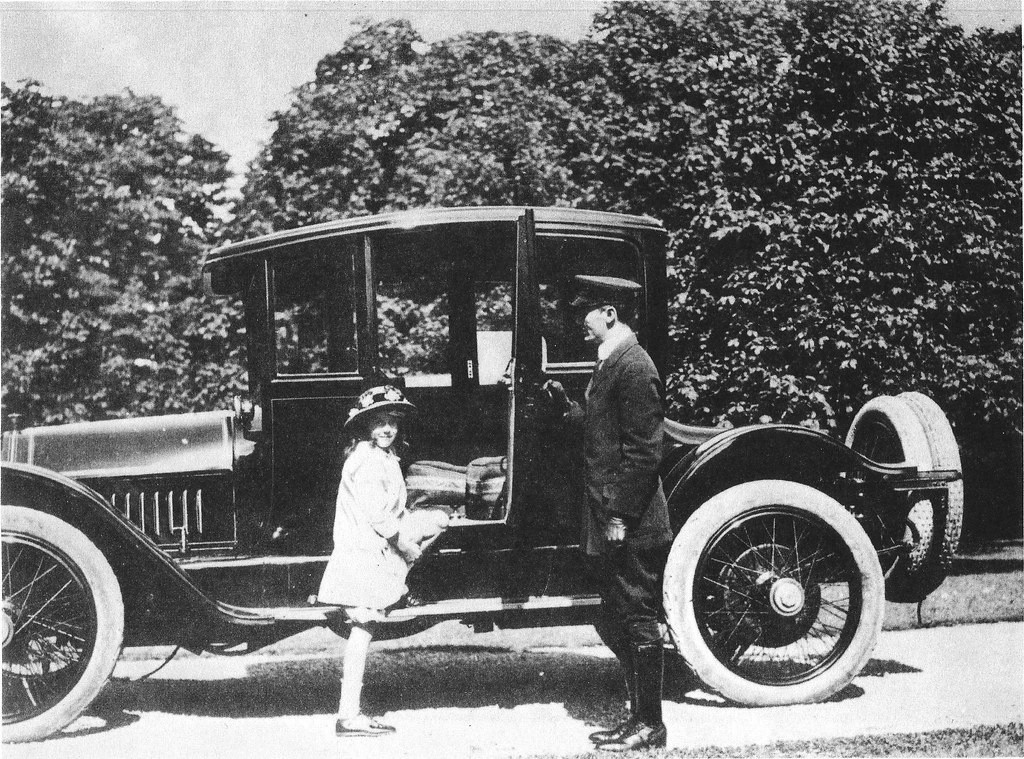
592,360,603,386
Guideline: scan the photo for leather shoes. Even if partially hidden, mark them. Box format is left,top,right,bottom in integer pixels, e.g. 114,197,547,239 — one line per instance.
589,715,666,753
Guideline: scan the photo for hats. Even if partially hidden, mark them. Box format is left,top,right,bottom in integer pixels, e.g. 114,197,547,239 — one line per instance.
343,386,416,429
572,275,642,306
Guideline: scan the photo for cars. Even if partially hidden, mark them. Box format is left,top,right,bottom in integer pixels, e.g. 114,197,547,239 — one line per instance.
1,205,968,742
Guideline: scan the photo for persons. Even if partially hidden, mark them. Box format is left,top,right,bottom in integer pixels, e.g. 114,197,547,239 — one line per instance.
316,384,419,735
543,273,673,753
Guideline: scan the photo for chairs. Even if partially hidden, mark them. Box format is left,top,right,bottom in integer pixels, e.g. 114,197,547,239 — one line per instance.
465,456,508,520
406,459,466,511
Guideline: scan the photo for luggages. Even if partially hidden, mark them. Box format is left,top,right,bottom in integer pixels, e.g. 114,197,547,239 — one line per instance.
465,456,507,520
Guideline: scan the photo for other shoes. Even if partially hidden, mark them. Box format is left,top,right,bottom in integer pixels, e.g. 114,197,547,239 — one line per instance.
336,710,396,737
